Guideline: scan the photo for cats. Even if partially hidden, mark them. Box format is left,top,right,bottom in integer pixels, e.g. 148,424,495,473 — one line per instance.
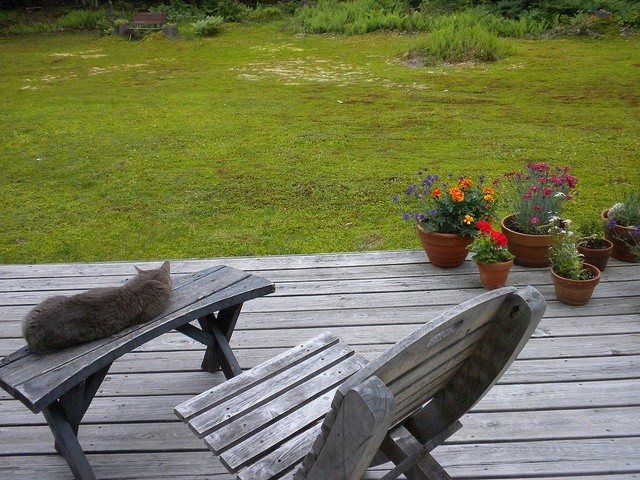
21,260,173,356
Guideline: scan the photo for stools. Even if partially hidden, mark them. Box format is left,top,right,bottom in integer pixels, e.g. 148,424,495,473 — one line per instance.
0,265,277,480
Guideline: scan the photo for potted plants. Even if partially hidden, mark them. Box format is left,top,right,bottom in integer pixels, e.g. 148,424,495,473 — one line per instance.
577,218,612,272
600,185,640,263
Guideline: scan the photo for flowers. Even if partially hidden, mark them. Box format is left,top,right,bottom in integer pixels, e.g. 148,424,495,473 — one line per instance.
469,221,512,263
502,162,578,227
392,169,501,235
546,213,585,280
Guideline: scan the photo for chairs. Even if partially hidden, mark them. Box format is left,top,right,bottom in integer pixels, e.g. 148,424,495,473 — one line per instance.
174,285,548,480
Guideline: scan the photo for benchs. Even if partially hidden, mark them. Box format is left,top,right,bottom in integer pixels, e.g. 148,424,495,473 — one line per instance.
124,12,166,40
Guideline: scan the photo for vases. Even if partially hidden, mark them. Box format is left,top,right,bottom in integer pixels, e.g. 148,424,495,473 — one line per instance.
476,258,513,290
551,263,600,306
417,222,474,268
502,213,567,267
